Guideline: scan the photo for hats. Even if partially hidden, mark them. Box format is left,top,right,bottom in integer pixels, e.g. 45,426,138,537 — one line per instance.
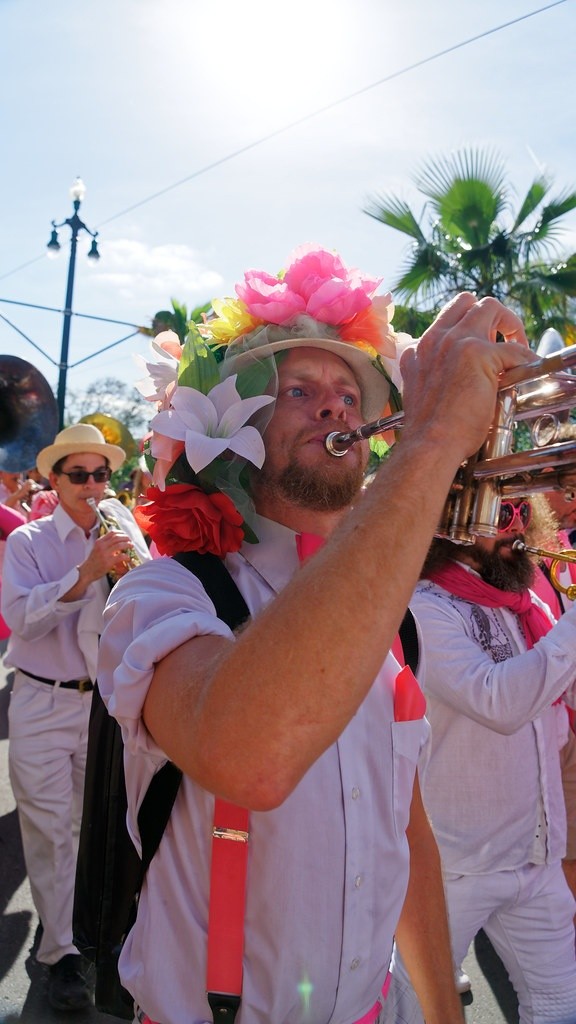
132,241,417,556
36,424,127,480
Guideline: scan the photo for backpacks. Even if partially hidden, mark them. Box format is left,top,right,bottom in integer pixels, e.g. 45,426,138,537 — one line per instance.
70,553,420,955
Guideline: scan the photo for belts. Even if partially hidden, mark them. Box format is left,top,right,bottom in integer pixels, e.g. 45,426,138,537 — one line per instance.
18,668,93,693
143,970,391,1024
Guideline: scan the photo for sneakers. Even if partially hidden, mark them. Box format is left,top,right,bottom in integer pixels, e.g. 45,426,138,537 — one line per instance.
48,954,92,1012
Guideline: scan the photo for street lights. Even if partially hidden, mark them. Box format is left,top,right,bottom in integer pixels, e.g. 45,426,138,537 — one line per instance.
45,172,101,432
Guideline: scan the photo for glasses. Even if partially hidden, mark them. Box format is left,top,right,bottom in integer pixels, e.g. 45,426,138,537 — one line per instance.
497,501,533,533
56,469,110,484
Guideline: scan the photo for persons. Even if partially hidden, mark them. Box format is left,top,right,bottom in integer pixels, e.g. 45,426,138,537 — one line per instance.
0,425,175,1012
94,293,544,1024
379,467,576,1024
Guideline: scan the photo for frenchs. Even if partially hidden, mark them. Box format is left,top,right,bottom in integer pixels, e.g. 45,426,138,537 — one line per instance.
1,353,61,512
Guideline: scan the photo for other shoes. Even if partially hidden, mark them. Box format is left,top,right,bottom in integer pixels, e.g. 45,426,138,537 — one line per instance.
459,967,472,992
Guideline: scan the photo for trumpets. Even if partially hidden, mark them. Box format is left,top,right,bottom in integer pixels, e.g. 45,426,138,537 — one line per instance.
325,345,576,549
84,496,141,583
511,538,576,600
